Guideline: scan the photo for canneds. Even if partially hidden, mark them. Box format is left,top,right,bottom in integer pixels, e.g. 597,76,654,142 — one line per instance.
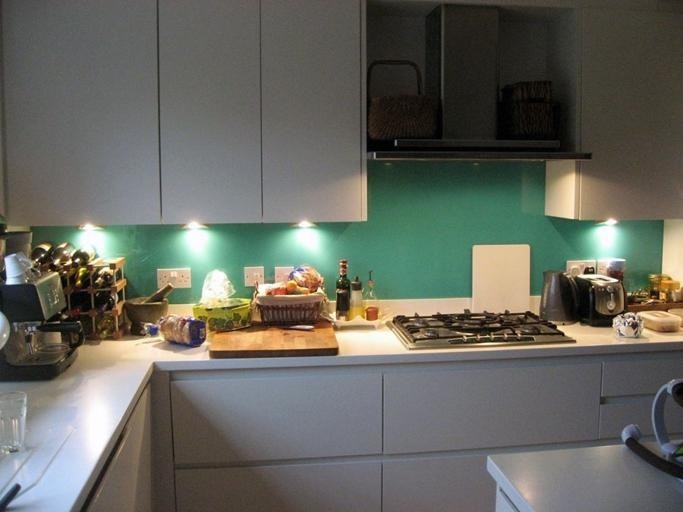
649,274,680,301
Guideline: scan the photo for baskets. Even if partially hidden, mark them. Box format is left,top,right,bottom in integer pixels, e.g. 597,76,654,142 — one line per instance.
256,283,326,325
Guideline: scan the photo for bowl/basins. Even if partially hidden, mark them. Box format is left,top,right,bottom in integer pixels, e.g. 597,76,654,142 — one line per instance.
122,293,170,331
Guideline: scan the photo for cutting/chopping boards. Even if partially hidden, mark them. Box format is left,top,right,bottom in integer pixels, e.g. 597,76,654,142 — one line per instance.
208,313,342,357
470,243,530,313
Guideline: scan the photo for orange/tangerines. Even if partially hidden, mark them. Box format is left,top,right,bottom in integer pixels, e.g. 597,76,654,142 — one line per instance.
287,280,297,292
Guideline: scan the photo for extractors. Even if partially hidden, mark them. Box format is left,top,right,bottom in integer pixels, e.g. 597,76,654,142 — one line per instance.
364,2,594,162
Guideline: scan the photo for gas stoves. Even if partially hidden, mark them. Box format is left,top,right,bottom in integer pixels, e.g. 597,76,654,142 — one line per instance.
384,308,580,349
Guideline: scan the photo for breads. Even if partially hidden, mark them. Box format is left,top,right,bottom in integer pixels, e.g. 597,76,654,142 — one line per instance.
162,315,203,346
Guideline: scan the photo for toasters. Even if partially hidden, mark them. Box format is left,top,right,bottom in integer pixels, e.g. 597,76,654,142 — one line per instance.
575,272,625,329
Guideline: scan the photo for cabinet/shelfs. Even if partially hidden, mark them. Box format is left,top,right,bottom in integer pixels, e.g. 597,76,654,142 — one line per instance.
152,356,382,512
44,254,127,342
0,0,159,230
158,1,368,227
78,368,155,512
598,341,681,446
381,346,601,511
541,1,681,222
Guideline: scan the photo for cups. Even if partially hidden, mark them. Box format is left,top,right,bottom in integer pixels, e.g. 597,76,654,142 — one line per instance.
1,391,31,455
1,251,42,285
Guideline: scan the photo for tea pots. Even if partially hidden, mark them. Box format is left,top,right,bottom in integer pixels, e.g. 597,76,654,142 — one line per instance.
537,268,581,326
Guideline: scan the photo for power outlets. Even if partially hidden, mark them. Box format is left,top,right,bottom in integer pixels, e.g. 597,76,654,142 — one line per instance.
154,267,192,289
563,257,596,276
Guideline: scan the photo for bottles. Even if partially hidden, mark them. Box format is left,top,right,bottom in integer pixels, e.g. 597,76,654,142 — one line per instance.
336,256,383,323
31,239,127,342
128,318,161,337
157,312,208,347
647,273,681,304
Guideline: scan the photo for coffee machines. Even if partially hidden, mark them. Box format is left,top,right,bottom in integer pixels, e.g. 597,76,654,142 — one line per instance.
1,260,81,382
